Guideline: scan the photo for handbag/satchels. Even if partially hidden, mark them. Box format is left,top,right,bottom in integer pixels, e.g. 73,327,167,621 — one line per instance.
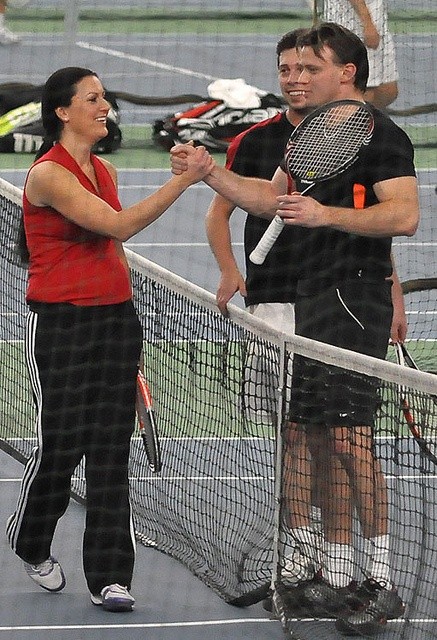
0,82,122,154
151,93,284,155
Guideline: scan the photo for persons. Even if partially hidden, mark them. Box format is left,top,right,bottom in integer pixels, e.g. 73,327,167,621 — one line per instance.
204,26,412,611
320,1,402,110
169,18,420,635
5,65,216,613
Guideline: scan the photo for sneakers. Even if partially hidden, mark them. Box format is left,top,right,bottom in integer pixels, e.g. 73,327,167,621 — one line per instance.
334,579,405,634
86,581,136,608
264,567,361,620
5,513,64,591
